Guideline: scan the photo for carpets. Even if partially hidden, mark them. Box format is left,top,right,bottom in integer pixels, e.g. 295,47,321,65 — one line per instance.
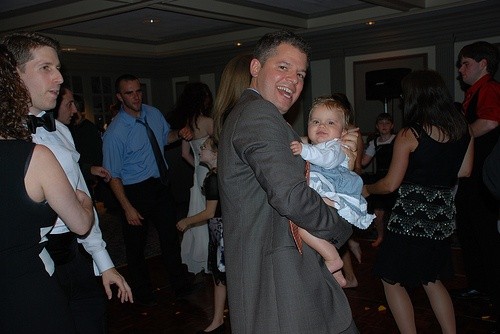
98,212,163,269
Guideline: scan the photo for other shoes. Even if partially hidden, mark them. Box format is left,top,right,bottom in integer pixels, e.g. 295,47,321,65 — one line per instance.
196,322,225,334
174,286,194,300
141,293,156,305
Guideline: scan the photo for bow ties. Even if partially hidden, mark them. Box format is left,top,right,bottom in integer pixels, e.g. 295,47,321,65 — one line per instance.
23,112,56,134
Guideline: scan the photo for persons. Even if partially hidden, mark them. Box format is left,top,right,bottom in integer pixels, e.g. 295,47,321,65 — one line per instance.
0,46,94,334
0,30,134,334
361,114,398,247
52,54,255,334
290,95,377,289
457,41,500,334
216,31,359,334
361,71,475,334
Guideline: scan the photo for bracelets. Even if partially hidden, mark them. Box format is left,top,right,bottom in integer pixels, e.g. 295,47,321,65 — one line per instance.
185,217,192,232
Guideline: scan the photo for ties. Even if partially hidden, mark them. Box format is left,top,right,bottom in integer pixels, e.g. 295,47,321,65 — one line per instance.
135,115,168,177
285,121,310,255
